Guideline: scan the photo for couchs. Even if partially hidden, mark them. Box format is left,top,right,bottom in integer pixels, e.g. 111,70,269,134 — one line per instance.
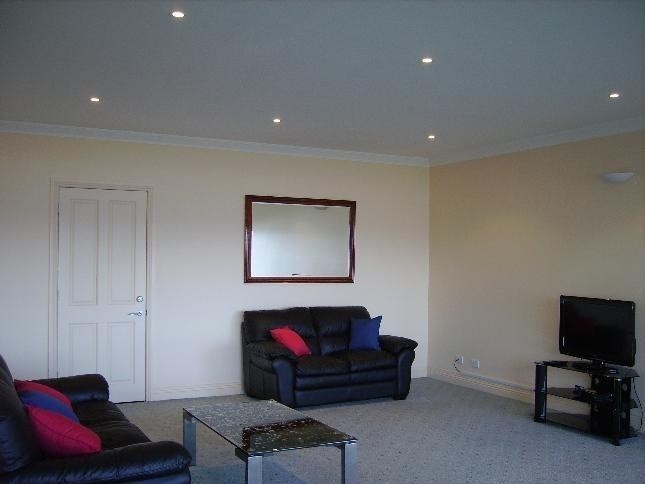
240,304,420,406
1,350,192,484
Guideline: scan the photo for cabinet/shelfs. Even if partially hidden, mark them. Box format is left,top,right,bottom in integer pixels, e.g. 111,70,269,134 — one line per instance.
533,360,641,446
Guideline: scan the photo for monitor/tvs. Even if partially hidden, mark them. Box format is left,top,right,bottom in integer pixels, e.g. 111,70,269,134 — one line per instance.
559,295,636,374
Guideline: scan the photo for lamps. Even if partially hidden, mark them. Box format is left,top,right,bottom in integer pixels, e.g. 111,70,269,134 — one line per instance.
602,170,634,183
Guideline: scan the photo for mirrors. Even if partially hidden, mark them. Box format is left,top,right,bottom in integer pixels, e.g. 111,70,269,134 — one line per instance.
244,194,356,282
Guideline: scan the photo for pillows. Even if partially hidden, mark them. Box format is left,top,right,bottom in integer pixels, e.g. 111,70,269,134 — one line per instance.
347,313,385,352
15,377,105,455
268,326,313,358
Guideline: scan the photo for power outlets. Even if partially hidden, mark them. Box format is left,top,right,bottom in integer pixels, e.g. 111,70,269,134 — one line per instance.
455,354,463,365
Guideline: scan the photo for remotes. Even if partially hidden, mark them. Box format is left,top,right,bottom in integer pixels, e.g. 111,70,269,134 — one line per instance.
582,388,599,400
